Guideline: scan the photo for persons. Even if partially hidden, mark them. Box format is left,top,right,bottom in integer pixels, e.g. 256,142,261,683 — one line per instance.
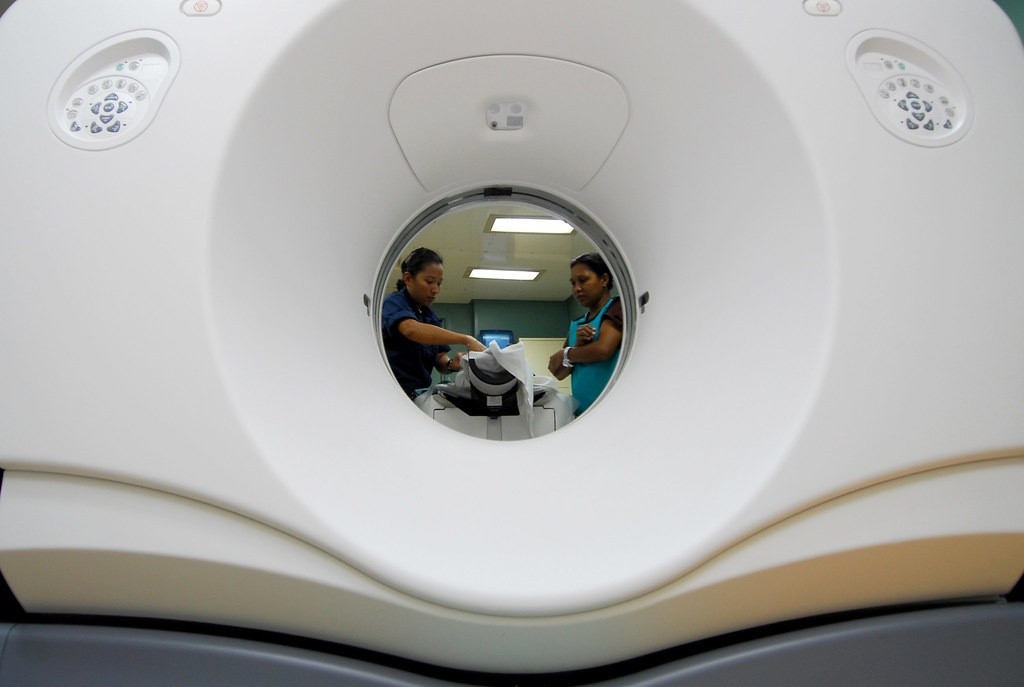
381,247,488,402
548,251,623,418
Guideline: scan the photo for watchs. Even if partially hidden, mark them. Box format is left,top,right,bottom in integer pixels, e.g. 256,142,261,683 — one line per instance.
447,359,460,372
563,347,575,367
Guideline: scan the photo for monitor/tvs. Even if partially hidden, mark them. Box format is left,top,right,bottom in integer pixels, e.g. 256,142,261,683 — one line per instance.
480,329,513,350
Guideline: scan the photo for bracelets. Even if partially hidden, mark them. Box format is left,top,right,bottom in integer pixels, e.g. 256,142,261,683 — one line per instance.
467,341,478,346
